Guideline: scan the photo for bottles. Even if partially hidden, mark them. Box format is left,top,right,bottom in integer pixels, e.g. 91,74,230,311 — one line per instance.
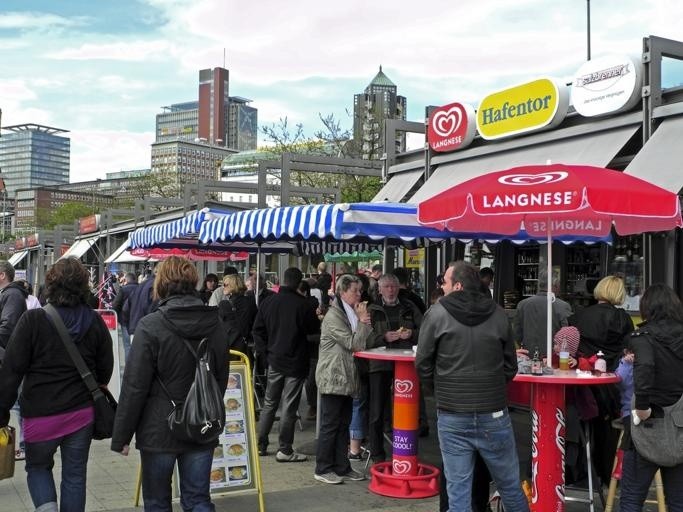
530,346,544,376
567,236,643,298
519,252,538,296
558,335,570,371
594,350,608,376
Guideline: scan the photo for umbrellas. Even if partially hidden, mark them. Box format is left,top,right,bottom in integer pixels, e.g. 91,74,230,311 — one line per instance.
416,160,683,369
199,200,614,275
127,208,404,309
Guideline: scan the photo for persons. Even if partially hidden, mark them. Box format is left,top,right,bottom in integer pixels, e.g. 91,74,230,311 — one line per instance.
1,253,683,512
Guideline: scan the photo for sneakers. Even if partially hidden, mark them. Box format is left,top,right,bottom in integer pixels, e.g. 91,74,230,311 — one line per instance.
258,450,269,457
346,451,363,462
313,470,343,484
342,469,366,481
275,448,307,462
304,406,316,420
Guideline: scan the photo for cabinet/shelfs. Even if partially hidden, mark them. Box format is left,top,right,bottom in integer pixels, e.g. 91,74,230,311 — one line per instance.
515,231,644,308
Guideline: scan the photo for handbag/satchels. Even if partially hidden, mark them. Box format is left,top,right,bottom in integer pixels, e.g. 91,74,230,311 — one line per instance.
0,423,18,481
88,385,119,440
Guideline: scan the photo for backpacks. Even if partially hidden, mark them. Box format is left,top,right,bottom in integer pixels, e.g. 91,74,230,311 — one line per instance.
144,305,228,447
628,389,682,469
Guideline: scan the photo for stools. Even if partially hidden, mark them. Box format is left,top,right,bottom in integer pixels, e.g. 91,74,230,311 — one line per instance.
604,417,669,512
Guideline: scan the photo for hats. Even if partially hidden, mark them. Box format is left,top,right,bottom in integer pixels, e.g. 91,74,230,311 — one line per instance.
552,325,580,355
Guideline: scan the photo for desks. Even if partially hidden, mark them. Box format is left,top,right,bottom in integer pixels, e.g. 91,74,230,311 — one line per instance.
513,366,621,512
351,346,442,498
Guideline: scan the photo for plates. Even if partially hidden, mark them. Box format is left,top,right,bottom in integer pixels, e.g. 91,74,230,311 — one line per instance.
209,446,247,483
225,374,242,433
